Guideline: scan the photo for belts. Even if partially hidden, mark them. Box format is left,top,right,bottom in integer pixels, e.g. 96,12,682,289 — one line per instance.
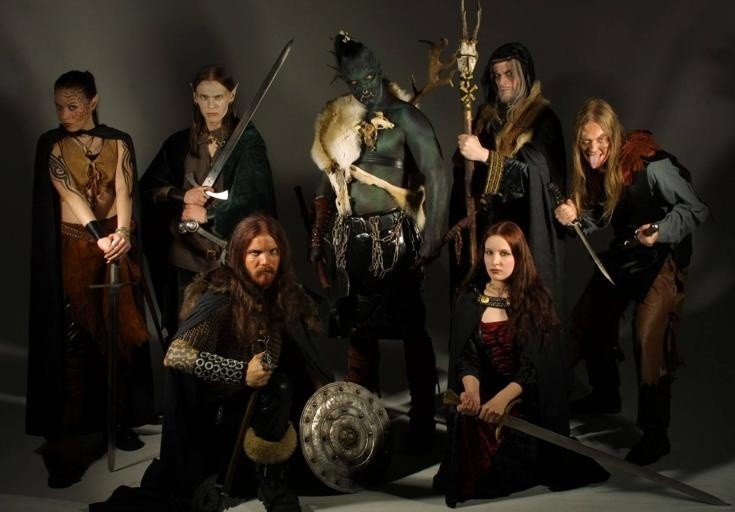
343,211,400,234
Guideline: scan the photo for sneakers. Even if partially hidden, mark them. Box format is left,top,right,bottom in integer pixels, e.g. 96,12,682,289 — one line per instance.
625,432,671,465
405,416,437,457
257,485,301,512
47,468,74,490
113,428,145,450
570,388,621,416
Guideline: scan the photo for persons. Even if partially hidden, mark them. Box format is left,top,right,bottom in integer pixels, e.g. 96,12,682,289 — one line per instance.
304,32,462,445
430,220,609,510
89,214,392,511
547,94,710,463
138,62,278,334
444,39,567,324
26,70,167,490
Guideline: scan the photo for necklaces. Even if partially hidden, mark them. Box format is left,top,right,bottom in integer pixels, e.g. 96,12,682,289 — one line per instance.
202,129,226,142
78,135,94,156
485,282,516,296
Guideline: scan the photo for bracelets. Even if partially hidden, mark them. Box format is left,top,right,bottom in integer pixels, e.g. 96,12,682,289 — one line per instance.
117,227,131,235
85,220,105,240
